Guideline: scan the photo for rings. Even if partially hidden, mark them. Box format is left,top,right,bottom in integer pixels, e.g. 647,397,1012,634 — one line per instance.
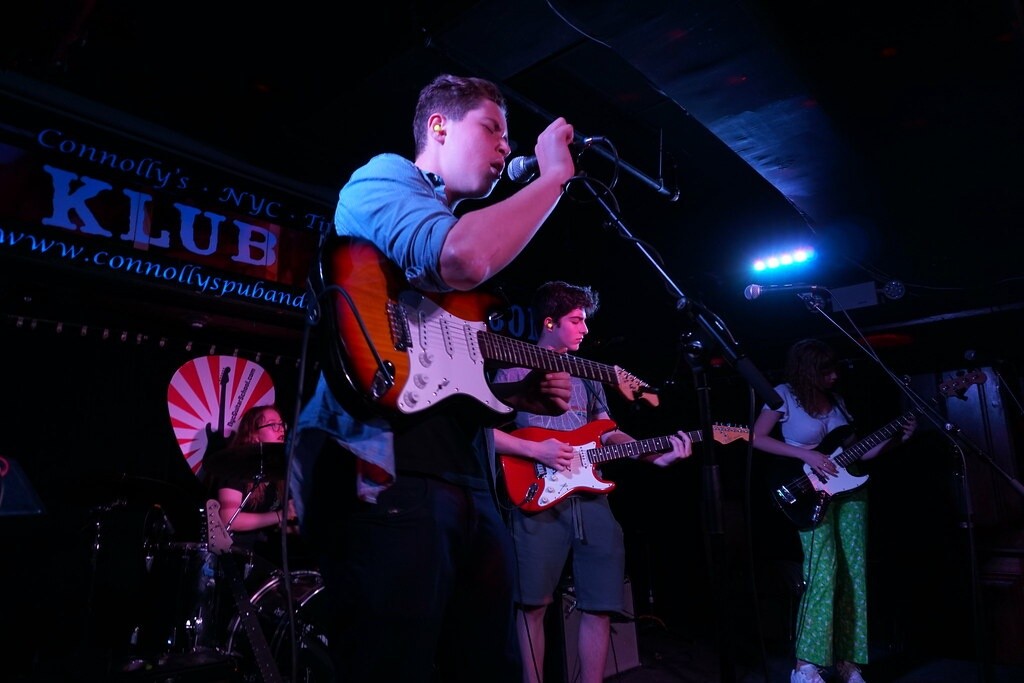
823,462,827,467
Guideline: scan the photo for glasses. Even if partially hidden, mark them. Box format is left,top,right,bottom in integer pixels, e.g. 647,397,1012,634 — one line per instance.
256,422,287,432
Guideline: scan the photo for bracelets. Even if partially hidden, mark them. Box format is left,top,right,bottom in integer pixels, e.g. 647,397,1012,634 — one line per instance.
275,511,280,524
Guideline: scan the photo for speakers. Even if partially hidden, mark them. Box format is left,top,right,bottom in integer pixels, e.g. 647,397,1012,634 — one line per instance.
541,570,643,683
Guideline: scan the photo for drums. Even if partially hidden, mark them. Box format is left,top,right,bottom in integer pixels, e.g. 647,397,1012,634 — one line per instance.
225,569,335,683
146,541,278,668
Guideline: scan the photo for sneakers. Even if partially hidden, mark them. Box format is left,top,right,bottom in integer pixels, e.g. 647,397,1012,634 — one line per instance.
836,660,866,683
790,663,825,683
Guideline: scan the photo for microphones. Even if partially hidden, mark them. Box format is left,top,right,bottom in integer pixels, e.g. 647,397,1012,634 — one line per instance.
744,283,828,302
155,505,175,536
506,135,604,182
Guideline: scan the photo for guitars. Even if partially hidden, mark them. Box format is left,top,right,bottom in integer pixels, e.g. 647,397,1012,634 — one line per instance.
308,235,661,434
197,496,285,682
498,418,752,512
761,366,988,530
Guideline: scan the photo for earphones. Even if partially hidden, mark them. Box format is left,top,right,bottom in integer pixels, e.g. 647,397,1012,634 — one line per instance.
547,323,553,329
434,125,442,132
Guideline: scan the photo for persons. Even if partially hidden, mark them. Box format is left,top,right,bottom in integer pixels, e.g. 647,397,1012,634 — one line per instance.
754,339,918,682
488,280,692,683
295,74,578,683
218,406,300,540
